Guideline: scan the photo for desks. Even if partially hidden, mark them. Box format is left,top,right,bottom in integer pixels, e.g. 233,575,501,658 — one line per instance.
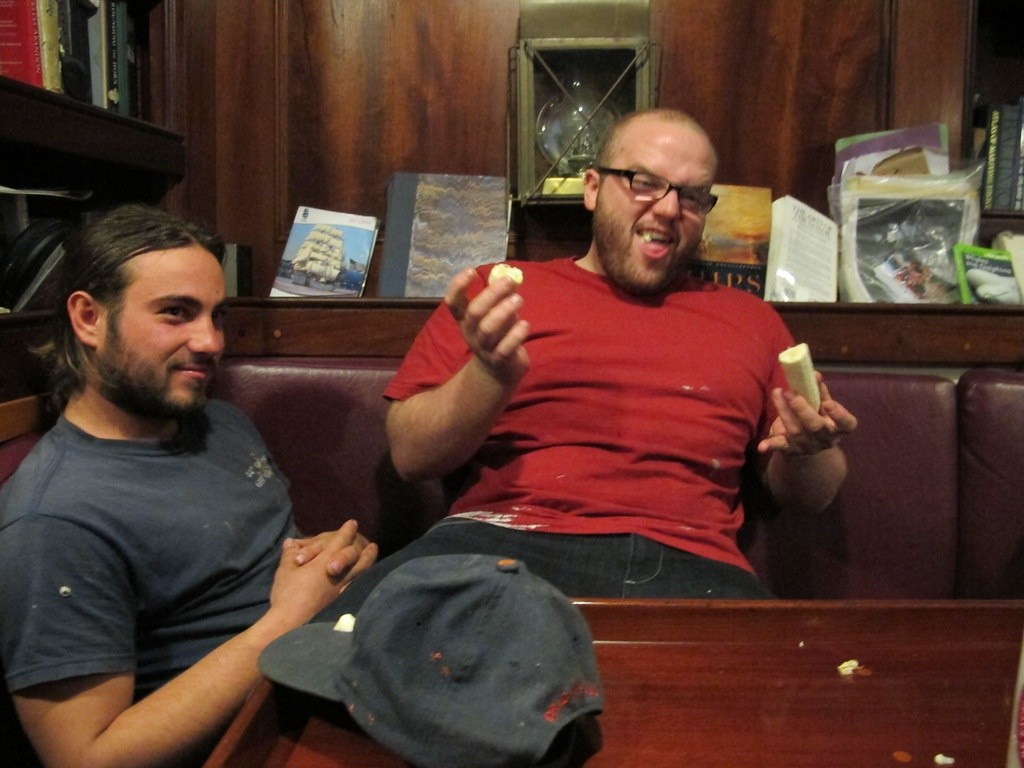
194,598,1024,768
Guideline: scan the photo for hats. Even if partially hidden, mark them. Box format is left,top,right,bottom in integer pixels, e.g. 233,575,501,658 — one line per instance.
257,553,603,768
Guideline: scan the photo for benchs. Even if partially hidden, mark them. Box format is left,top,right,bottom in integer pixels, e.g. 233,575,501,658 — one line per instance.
0,365,1023,605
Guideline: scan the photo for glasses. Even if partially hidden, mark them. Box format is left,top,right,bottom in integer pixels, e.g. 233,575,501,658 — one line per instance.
598,166,718,214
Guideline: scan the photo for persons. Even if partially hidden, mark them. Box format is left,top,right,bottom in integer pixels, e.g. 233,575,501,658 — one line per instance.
306,108,858,635
0,204,379,768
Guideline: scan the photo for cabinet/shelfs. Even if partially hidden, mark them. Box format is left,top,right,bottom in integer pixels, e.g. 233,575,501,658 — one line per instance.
0,74,189,403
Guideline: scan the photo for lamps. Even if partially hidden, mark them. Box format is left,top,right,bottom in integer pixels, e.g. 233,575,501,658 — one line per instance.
537,46,624,181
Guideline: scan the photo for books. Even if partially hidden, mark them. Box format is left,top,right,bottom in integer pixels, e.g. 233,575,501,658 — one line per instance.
764,195,836,303
954,245,1020,306
697,185,774,295
270,205,380,297
379,172,511,300
983,99,1024,212
0,0,153,314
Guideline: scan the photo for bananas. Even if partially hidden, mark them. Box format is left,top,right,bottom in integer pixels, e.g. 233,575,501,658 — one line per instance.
778,343,821,413
488,264,523,287
